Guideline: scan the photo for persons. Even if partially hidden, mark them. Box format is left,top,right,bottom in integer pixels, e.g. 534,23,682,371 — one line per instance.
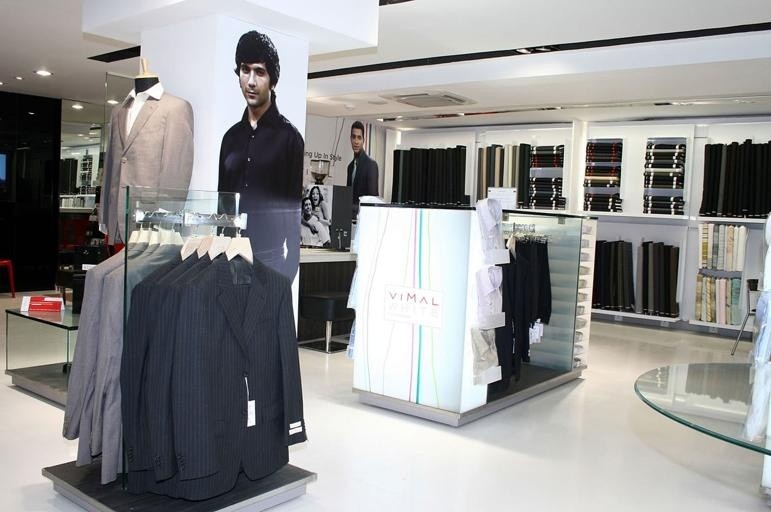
96,71,195,246
299,197,328,247
215,28,304,287
345,118,379,224
299,185,330,246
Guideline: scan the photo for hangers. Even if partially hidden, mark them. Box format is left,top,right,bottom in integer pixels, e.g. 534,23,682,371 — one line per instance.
128,210,254,266
503,222,553,244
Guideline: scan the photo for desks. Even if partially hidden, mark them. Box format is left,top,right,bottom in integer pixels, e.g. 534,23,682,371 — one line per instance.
299,244,358,264
4,304,80,407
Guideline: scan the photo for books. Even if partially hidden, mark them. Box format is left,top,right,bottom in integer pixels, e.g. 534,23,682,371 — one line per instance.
19,295,63,313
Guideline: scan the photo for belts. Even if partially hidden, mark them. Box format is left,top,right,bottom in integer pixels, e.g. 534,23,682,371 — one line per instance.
254,245,285,261
352,219,357,225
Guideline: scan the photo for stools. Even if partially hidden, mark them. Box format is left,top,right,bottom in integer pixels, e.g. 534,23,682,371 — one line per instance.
0,259,16,298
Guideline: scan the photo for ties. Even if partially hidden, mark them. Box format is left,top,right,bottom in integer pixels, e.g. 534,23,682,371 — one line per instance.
350,159,357,192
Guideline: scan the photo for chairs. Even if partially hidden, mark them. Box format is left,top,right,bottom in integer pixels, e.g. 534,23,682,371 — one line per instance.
731,278,761,356
